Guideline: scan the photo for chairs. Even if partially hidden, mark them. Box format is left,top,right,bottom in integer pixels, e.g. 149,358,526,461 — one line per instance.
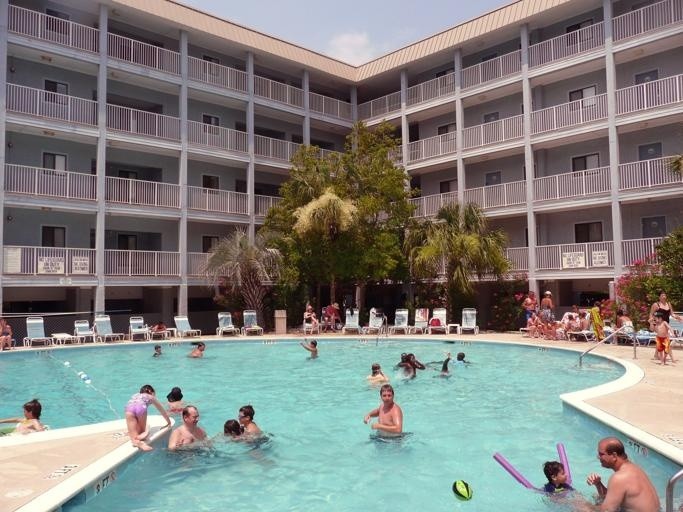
0,310,263,350
302,307,479,336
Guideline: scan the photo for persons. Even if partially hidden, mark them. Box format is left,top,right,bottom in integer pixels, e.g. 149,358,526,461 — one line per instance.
304,305,319,334
300,338,317,357
188,342,205,358
125,385,266,452
366,351,466,384
363,384,403,439
323,302,341,333
585,437,661,512
522,290,633,345
149,322,166,332
152,345,162,356
650,291,680,362
543,461,576,493
0,318,13,351
0,399,49,436
649,312,674,366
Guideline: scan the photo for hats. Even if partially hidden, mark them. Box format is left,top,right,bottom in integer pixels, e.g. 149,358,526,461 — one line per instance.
543,290,552,296
331,302,340,310
310,340,317,347
653,311,663,317
305,305,314,311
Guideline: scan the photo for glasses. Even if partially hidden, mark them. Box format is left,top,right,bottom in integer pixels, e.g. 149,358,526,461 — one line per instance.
237,416,247,420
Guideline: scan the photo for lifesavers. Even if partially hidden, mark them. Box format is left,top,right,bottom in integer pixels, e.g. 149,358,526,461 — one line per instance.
1,425,50,435
393,363,413,382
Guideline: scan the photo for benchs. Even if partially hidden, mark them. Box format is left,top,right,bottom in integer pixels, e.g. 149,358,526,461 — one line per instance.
519,312,683,347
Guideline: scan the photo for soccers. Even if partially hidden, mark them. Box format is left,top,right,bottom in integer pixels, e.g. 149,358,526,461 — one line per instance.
451,479,474,501
